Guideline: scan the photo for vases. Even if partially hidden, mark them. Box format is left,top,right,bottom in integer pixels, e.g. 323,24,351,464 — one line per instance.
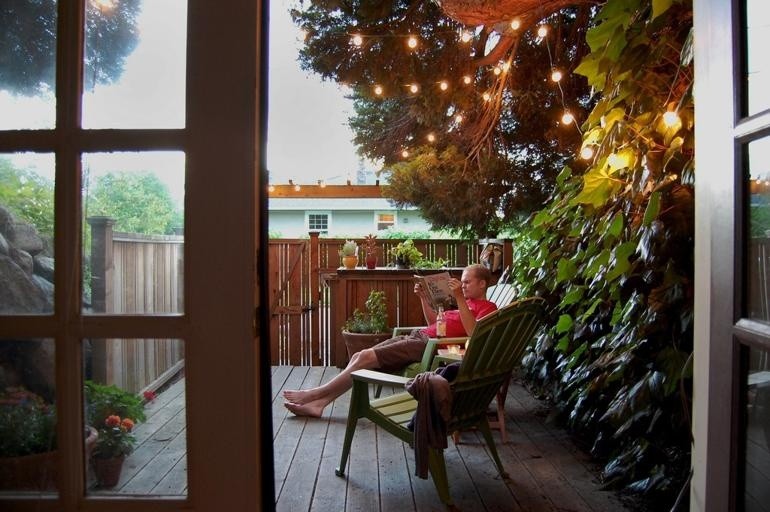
0,427,98,491
92,453,125,489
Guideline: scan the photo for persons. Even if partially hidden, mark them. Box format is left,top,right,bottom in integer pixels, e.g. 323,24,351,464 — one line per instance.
281,264,499,420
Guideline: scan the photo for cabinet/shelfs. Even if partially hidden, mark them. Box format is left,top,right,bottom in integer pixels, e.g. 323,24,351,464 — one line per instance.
336,266,501,369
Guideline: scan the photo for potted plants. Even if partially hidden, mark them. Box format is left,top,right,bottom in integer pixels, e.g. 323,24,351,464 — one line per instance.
338,234,449,270
340,288,393,365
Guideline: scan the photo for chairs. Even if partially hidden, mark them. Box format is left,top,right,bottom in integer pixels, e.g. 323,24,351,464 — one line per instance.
335,297,557,510
374,284,518,400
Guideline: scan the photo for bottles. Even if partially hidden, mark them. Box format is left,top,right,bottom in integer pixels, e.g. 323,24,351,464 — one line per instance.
436,306,447,339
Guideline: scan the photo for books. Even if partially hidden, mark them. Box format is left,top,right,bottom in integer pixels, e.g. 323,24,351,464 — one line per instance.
410,271,461,312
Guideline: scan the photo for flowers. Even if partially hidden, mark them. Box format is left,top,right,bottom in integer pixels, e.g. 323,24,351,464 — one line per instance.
0,380,157,459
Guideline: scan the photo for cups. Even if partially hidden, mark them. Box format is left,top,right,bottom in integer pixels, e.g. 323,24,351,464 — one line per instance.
448,345,460,359
